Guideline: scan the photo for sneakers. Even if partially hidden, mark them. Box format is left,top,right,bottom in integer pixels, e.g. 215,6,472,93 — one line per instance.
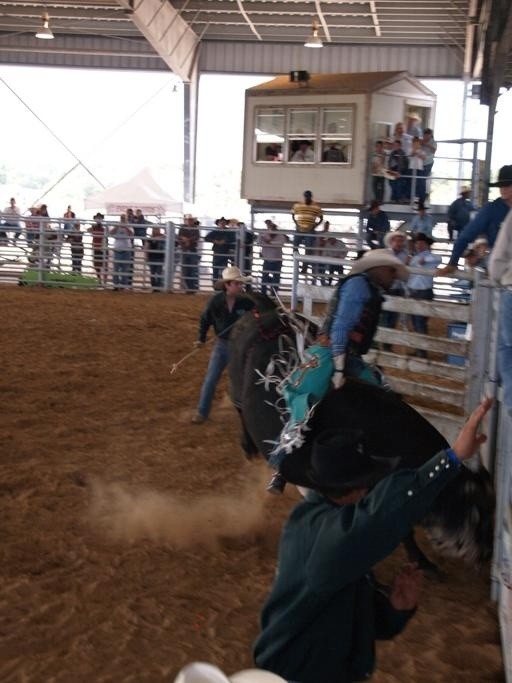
191,412,205,424
265,473,287,495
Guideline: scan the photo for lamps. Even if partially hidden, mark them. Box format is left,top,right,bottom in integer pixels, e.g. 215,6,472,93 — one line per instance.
35,11,56,40
302,18,325,48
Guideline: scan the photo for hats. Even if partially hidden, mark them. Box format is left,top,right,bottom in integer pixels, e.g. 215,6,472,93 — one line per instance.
407,111,422,122
459,186,473,195
215,217,239,227
278,426,391,489
366,199,384,212
345,248,410,282
383,231,408,247
413,202,430,210
181,213,198,220
410,233,434,245
381,169,401,181
485,165,512,186
216,265,252,284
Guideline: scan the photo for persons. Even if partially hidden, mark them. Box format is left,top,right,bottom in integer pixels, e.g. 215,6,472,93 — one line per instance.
2,186,509,357
262,109,437,204
267,249,410,493
433,164,511,276
254,390,493,682
192,263,274,423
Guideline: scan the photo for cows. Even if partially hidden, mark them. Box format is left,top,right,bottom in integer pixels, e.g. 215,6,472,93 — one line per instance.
228,308,496,580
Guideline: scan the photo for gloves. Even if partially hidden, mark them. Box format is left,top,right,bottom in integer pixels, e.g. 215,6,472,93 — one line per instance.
331,353,347,392
